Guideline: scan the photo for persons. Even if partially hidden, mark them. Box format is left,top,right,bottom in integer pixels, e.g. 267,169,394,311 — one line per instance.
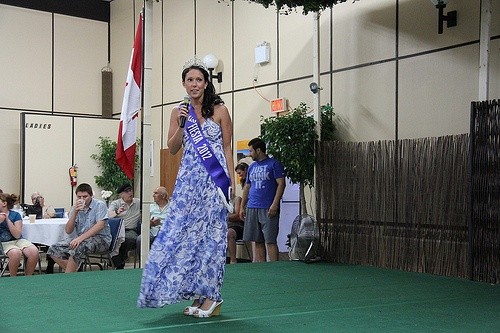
227,195,246,264
0,189,23,211
47,183,112,273
0,193,38,276
137,185,170,256
239,138,286,262
108,183,140,269
235,162,250,191
30,194,55,219
137,55,236,317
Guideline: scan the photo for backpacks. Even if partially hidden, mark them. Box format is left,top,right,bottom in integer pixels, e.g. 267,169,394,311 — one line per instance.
289,214,321,261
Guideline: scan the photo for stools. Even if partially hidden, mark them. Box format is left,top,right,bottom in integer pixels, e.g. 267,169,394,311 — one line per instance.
236,240,252,263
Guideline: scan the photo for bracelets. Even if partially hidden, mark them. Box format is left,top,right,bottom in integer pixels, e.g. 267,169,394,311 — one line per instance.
115,208,119,215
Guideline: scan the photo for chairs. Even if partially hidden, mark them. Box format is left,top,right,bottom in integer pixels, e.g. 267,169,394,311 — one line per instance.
81,218,123,272
0,239,42,278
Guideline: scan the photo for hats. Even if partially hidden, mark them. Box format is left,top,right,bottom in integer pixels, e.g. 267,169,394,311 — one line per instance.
116,183,133,194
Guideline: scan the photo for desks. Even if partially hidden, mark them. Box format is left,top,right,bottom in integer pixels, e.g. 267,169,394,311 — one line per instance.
21,218,125,274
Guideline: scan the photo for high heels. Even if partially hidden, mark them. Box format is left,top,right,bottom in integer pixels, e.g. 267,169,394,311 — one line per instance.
184,299,205,315
193,299,224,318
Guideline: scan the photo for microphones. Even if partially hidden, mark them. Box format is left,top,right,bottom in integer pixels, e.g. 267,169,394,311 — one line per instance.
179,96,191,128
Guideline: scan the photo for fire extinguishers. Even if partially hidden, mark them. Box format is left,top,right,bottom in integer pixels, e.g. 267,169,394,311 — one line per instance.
69,163,78,186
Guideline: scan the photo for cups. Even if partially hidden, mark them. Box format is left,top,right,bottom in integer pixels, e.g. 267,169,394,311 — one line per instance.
29,214,37,223
77,199,85,209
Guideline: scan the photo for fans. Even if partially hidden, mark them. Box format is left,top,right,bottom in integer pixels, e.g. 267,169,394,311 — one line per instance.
291,214,321,263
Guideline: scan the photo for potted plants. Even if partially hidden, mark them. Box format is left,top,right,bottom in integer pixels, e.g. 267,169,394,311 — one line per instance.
285,234,297,259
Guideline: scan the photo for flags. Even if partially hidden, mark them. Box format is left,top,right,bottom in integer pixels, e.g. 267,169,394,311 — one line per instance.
114,9,143,180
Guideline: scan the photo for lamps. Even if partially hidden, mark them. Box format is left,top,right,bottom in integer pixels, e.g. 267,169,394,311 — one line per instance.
431,0,457,35
310,83,318,94
202,54,222,83
